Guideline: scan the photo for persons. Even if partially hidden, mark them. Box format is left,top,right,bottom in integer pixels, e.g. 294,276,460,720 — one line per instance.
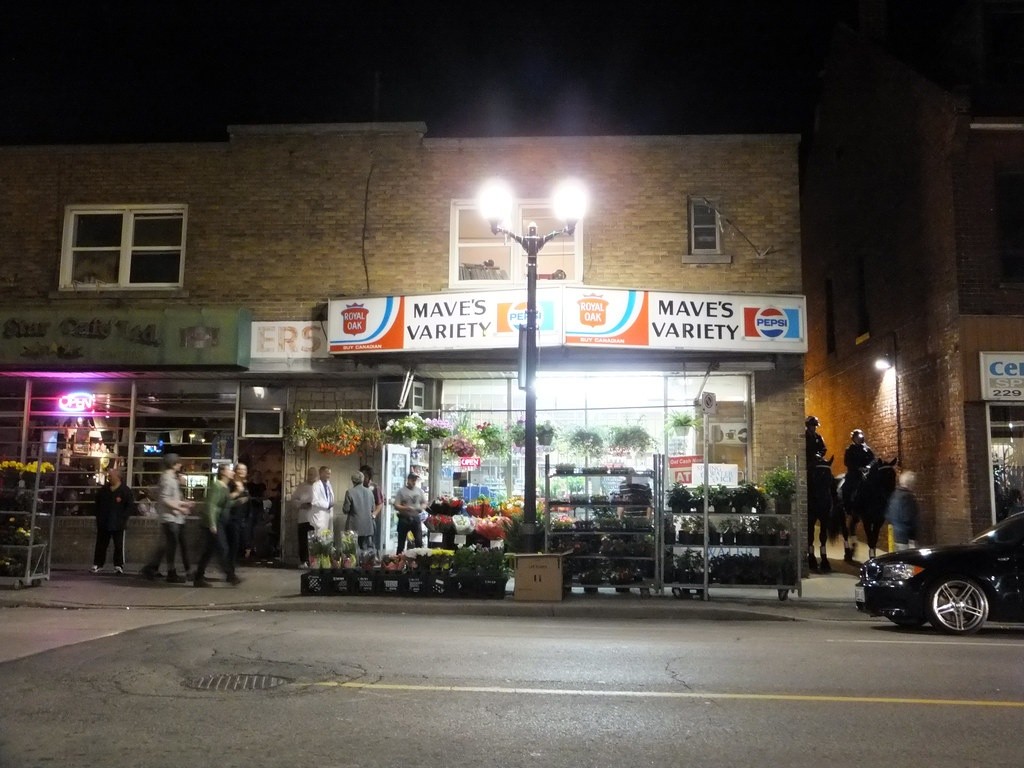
393,472,426,555
805,416,827,457
342,466,383,553
885,471,919,550
291,466,334,568
88,468,134,574
137,452,267,588
841,429,874,515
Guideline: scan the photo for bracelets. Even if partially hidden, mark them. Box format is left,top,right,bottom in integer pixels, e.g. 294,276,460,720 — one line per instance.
372,514,376,519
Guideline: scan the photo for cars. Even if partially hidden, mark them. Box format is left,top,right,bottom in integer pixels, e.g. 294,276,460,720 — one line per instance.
853,508,1024,636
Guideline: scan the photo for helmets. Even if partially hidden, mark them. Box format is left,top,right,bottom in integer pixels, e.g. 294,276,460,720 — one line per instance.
851,429,863,440
805,414,821,427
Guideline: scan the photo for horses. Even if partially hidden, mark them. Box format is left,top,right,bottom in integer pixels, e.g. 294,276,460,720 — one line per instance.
809,453,834,571
831,457,897,561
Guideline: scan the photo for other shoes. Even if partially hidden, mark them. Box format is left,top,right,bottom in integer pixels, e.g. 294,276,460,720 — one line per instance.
167,568,186,583
141,564,164,581
298,561,308,569
226,573,241,582
194,579,212,587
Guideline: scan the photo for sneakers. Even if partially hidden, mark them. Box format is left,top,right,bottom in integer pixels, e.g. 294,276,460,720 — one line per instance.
114,565,123,575
89,565,103,573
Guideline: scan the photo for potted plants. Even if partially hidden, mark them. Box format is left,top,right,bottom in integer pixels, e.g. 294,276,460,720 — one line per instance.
688,482,717,514
578,568,603,593
668,409,703,436
607,565,638,593
450,546,514,601
732,481,760,514
673,514,797,585
765,467,797,515
712,483,737,513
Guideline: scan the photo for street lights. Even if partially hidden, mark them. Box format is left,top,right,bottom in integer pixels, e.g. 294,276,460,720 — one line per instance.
474,175,588,555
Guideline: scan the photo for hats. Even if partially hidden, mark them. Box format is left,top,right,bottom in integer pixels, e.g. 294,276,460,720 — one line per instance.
163,453,179,465
408,472,420,478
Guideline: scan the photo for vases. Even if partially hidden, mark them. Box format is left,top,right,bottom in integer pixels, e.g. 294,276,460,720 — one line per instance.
456,450,475,457
402,434,418,449
4,473,17,488
515,440,525,447
294,435,308,446
431,437,442,448
23,472,38,488
539,438,552,445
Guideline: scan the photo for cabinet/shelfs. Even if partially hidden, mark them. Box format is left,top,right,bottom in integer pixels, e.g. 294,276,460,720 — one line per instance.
544,450,660,597
0,446,60,586
663,454,802,602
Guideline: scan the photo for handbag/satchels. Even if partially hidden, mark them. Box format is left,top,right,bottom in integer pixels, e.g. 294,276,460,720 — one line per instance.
263,499,272,513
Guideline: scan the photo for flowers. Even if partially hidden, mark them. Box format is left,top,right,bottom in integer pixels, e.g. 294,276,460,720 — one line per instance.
287,413,563,460
0,459,56,474
304,528,456,570
